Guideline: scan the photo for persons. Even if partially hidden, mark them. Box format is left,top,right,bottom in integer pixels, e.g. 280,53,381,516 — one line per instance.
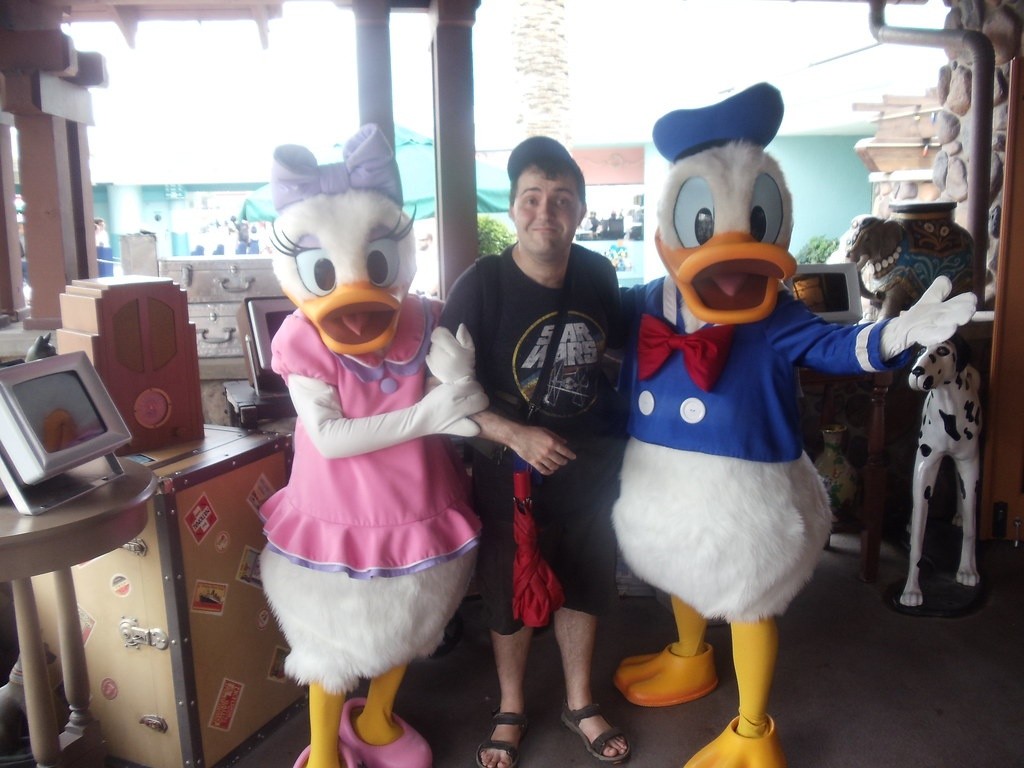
589,209,624,232
94,218,111,247
426,135,632,768
18,222,28,284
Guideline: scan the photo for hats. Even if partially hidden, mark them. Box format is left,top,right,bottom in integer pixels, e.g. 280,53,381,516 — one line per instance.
507,135,586,202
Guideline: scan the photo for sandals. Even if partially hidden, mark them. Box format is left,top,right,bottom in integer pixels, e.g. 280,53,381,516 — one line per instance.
475,706,529,768
561,702,631,762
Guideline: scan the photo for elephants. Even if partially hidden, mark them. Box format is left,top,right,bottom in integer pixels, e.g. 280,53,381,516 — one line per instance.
844,216,974,322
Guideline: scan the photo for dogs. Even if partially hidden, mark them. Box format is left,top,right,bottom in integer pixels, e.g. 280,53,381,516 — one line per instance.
900,338,981,606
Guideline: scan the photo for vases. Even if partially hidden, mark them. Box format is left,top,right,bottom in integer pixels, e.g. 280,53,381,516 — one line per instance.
812,423,860,524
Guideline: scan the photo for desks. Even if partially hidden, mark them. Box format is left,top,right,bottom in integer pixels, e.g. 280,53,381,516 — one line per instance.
0,457,158,768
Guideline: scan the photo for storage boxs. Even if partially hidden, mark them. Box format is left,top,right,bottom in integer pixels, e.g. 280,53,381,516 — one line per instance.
155,253,285,358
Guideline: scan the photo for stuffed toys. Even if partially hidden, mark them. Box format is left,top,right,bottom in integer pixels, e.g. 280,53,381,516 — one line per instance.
258,122,489,768
618,80,977,768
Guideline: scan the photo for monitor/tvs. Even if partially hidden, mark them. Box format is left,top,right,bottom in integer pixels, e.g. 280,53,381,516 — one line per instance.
793,262,865,327
237,295,300,389
0,349,133,500
